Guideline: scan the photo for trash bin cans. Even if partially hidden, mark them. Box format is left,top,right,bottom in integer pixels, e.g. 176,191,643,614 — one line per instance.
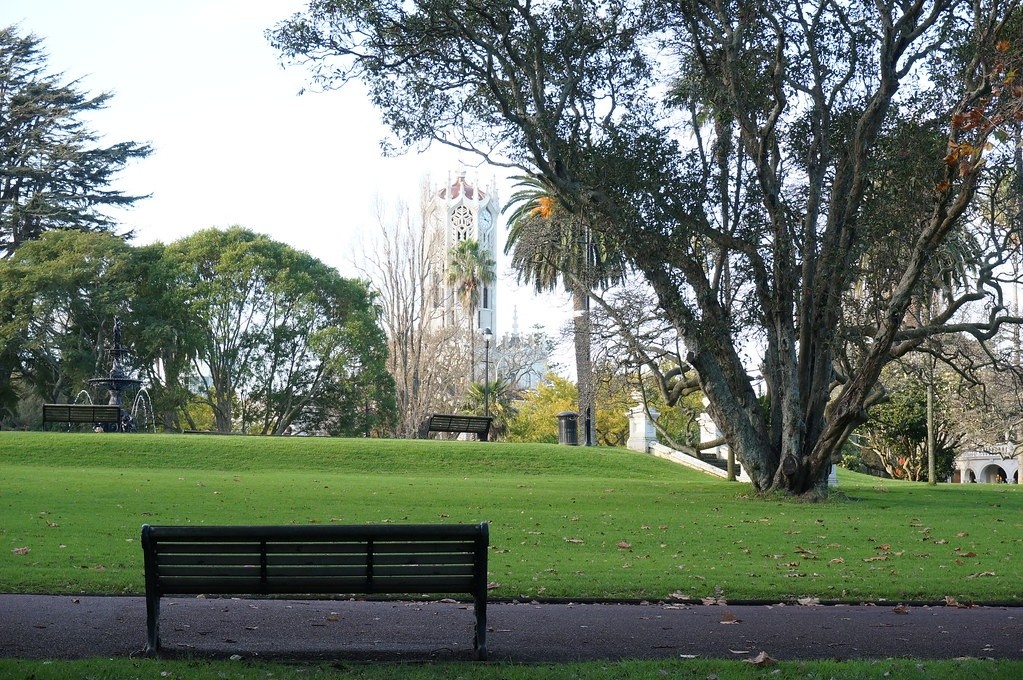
556,412,579,445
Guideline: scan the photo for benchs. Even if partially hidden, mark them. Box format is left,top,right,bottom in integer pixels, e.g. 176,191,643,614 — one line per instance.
42,403,122,433
418,414,493,442
141,522,489,656
